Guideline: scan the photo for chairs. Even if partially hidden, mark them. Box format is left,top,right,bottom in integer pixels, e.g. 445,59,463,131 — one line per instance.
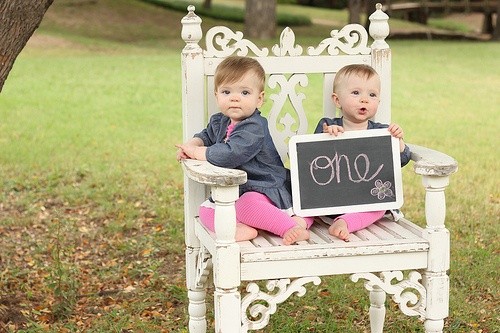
181,3,459,333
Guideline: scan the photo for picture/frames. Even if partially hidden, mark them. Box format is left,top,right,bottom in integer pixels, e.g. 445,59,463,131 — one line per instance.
289,128,404,217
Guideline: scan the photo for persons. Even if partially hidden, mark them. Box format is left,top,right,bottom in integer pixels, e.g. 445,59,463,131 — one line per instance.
289,64,411,242
173,56,311,247
